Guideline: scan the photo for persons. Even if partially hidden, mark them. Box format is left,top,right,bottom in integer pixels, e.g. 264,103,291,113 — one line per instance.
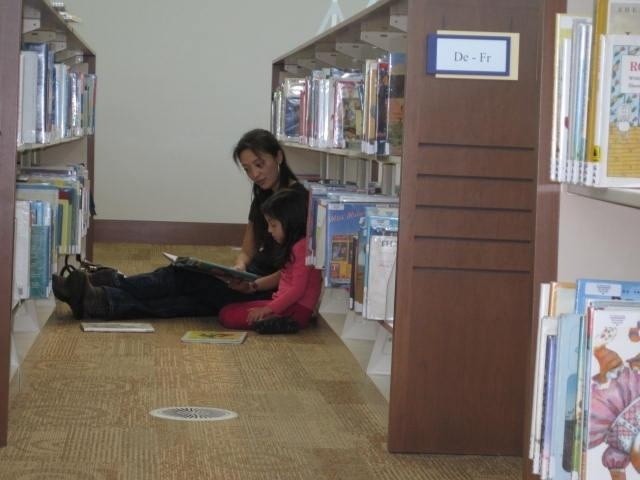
216,186,323,333
53,128,309,323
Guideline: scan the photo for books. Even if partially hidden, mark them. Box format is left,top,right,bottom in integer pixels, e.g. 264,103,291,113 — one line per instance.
181,329,249,344
10,41,94,318
163,251,261,282
549,0,639,189
524,275,639,479
270,49,407,325
82,322,155,334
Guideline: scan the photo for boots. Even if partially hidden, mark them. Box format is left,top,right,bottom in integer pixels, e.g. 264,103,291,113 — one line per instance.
52,266,120,320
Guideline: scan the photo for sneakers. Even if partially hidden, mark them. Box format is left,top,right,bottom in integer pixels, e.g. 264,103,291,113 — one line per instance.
254,314,299,334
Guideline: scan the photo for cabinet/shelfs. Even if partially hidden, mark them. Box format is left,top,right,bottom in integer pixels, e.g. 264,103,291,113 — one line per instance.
0,0,98,449
